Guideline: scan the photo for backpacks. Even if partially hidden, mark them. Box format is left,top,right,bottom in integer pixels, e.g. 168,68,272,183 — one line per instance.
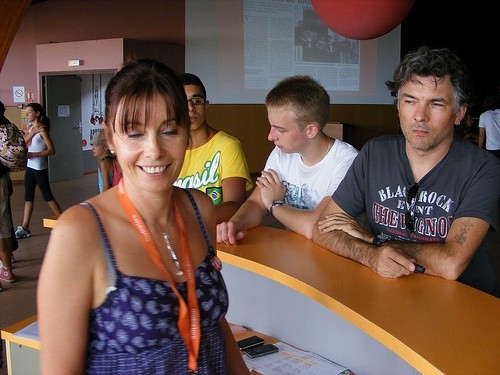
0,123,27,170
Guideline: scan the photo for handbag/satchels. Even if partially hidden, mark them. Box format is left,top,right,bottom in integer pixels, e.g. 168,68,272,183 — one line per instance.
22,131,32,147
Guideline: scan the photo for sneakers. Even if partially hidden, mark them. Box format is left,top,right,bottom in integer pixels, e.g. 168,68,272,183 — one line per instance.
14,227,32,238
0,264,16,284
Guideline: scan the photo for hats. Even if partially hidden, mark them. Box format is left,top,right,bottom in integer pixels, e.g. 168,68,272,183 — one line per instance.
89,131,105,146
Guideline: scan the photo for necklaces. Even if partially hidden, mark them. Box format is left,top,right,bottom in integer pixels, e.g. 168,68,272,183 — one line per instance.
115,175,202,375
137,209,184,275
323,136,331,157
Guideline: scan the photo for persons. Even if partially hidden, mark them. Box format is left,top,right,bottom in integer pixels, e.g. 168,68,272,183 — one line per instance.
313,46,500,281
172,73,254,224
14,103,62,239
0,100,26,291
90,132,123,194
36,54,251,375
217,73,360,246
455,96,500,155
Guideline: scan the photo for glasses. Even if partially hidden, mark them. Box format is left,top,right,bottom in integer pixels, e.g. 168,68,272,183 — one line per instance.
405,183,420,232
184,97,205,106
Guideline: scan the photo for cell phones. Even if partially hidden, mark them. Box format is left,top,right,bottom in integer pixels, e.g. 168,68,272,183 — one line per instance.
236,336,265,351
244,344,279,359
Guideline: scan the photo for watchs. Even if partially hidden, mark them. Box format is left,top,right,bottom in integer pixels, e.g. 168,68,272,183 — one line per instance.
373,231,392,247
270,200,286,217
